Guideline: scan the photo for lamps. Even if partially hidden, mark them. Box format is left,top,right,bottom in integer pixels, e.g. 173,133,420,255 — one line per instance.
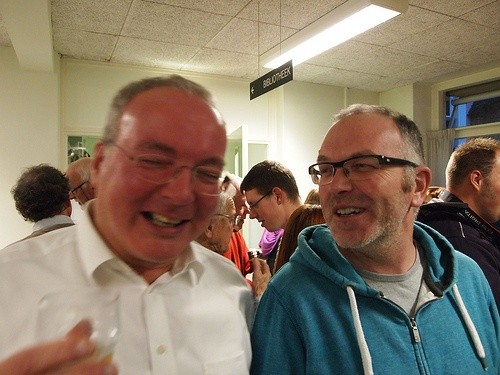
260,0,410,69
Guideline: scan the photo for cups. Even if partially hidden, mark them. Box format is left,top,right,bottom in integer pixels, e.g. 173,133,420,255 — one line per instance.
36,287,122,370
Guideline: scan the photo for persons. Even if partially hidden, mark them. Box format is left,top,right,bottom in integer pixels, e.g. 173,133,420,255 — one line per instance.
11,163,77,244
63,158,96,205
0,74,258,375
193,160,327,307
414,136,500,309
249,103,500,375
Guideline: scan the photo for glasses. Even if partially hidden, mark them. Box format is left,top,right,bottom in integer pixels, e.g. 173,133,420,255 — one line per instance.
308,154,420,184
249,191,272,213
106,140,232,196
69,181,87,197
215,213,242,226
